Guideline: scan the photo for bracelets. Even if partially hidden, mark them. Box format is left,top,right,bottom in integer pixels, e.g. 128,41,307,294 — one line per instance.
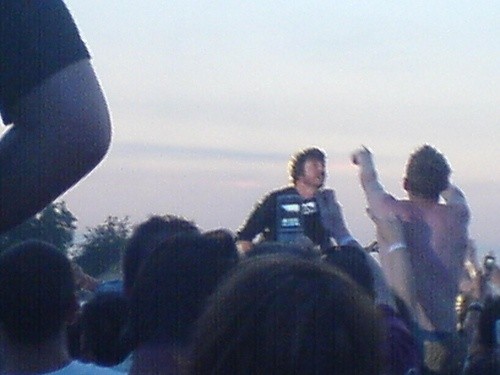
386,243,408,254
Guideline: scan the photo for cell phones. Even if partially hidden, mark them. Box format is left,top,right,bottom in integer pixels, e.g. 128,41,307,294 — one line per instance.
484,256,496,277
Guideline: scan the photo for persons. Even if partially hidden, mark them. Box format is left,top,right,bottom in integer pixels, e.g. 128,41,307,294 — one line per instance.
237,148,353,252
351,144,471,374
0,214,500,375
0,0,113,236
